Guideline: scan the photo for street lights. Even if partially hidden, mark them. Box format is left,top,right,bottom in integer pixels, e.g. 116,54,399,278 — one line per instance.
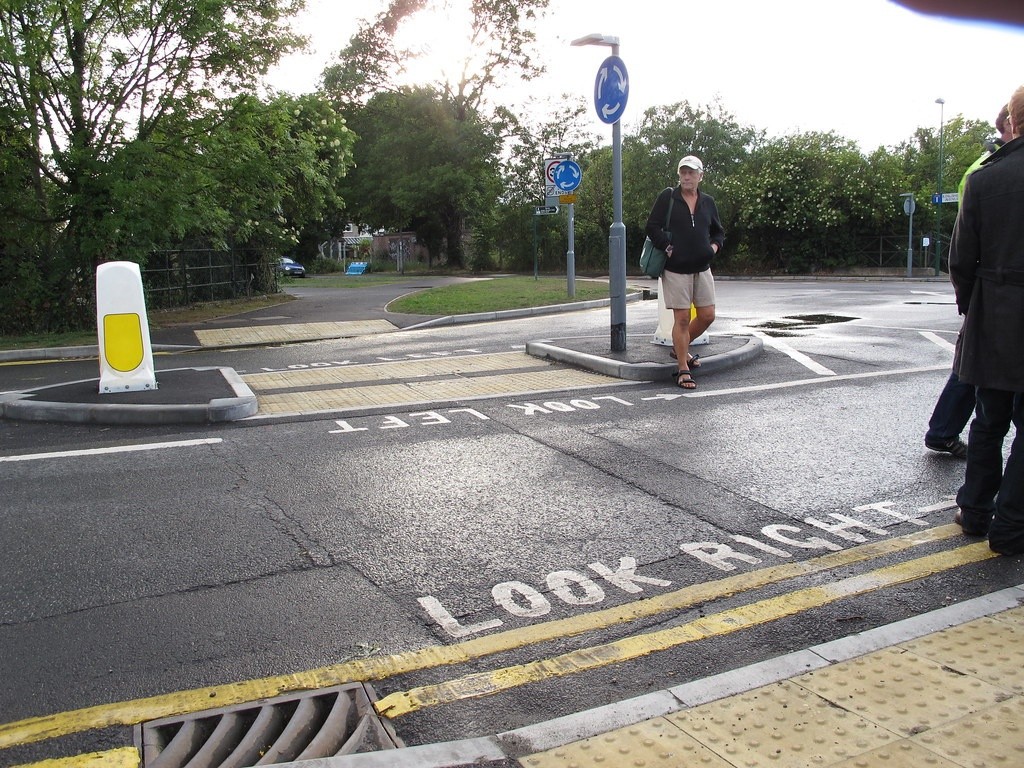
570,32,632,353
934,98,945,276
899,188,916,276
556,149,583,296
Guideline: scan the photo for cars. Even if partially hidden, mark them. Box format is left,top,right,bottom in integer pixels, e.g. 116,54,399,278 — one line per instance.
277,257,306,278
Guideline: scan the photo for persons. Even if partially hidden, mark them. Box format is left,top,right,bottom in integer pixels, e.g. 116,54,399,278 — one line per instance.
645,155,726,388
955,85,1024,558
926,111,1012,458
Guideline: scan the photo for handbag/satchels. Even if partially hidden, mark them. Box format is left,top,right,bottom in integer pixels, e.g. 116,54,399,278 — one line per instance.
639,187,674,277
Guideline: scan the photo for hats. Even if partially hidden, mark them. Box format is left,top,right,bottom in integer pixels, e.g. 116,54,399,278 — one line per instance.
678,155,703,170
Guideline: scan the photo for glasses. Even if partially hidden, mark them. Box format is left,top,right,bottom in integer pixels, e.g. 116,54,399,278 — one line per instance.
1007,115,1011,125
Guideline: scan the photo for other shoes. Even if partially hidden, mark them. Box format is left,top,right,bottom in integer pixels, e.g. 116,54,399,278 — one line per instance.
990,542,1024,555
955,507,988,537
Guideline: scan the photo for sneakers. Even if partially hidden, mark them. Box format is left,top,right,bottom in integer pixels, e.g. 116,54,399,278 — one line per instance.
925,434,968,459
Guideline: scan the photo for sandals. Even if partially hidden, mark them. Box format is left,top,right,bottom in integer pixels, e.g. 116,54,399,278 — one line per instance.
670,347,701,368
675,370,697,389
